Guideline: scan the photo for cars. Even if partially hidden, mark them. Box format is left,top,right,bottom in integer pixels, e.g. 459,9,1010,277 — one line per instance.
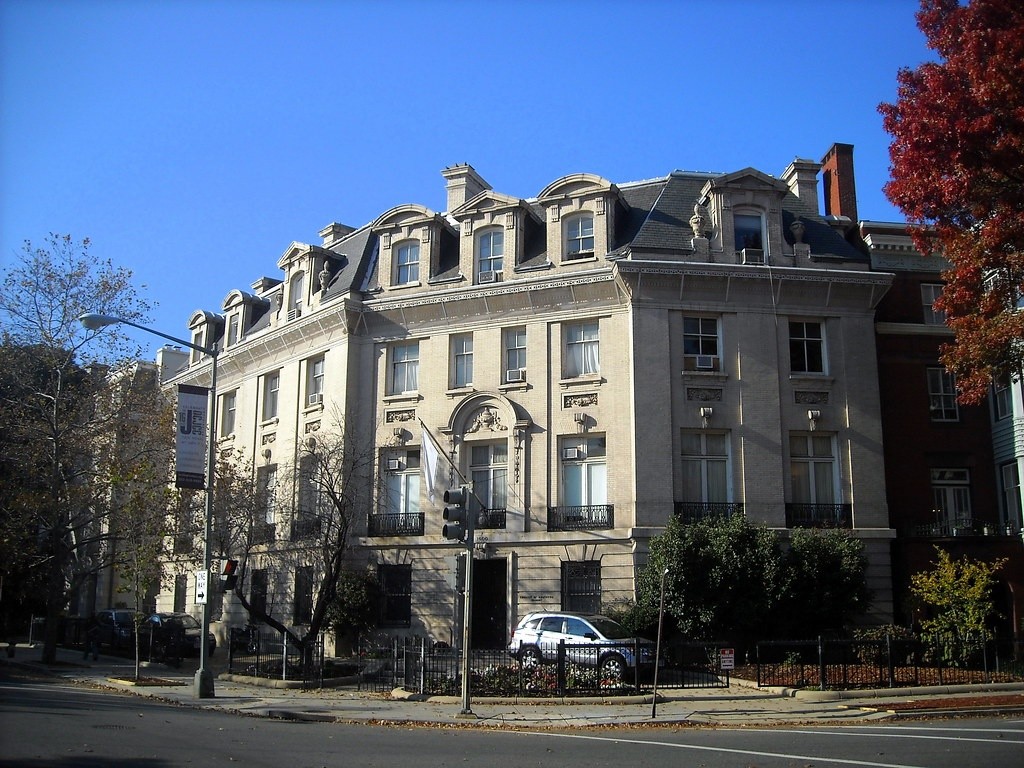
95,609,150,656
137,612,217,660
230,625,257,656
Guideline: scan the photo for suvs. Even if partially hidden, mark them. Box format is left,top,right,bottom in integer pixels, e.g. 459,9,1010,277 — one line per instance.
506,611,670,682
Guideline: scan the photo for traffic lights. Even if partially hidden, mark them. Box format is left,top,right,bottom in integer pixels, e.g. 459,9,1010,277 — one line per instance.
220,559,238,575
443,487,465,541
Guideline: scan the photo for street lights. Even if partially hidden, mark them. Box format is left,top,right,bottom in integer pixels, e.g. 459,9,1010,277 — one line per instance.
79,312,215,700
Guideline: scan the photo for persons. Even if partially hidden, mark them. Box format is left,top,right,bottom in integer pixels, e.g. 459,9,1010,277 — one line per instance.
83,610,101,661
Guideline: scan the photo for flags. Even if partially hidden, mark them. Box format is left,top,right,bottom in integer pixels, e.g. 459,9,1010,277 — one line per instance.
420,428,439,507
